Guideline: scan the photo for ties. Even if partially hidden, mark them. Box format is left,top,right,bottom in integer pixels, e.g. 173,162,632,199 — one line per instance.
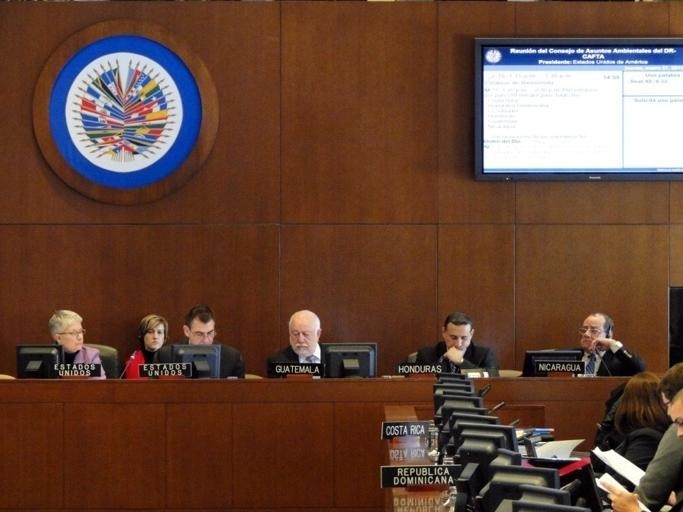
586,352,596,374
306,356,319,363
450,363,457,373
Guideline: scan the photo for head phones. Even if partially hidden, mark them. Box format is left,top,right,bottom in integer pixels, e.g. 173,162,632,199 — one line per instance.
606,314,614,339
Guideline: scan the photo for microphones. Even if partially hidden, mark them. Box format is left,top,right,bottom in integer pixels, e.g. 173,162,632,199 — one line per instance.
479,384,491,397
555,478,581,493
508,418,520,426
517,431,532,440
489,400,505,415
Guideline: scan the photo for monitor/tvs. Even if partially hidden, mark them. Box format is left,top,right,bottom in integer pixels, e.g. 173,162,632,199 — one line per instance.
559,462,604,512
523,348,582,377
440,373,467,380
172,343,221,378
441,406,488,424
16,344,64,378
446,411,499,456
433,384,472,395
443,391,477,397
488,464,559,511
523,437,537,457
473,36,682,184
513,501,592,511
476,448,521,511
434,395,484,413
518,484,571,506
439,379,471,385
528,458,582,469
454,422,519,512
320,342,377,378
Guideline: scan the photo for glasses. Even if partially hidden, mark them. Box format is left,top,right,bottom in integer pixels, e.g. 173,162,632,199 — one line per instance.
190,330,217,338
579,328,608,335
56,329,86,337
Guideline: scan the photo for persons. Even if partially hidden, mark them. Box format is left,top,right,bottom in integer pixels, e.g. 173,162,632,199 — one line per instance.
266,309,343,378
428,311,500,374
123,314,169,381
170,304,245,379
589,361,682,511
569,312,645,378
49,309,106,381
